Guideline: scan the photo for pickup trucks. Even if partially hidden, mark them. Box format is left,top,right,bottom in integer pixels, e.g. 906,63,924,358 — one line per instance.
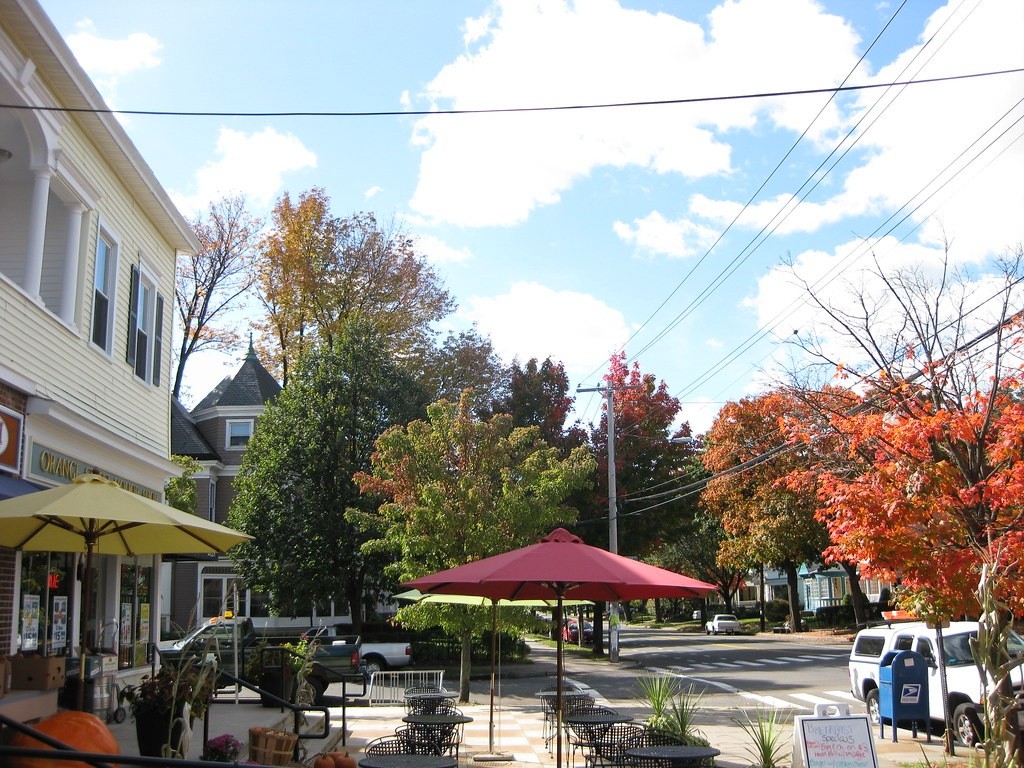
298,627,412,679
160,610,366,709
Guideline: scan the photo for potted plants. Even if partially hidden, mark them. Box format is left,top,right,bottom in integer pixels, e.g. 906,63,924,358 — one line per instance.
118,660,217,760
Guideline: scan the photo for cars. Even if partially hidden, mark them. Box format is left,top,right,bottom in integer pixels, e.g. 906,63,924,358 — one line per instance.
531,611,569,641
705,614,740,635
563,619,593,645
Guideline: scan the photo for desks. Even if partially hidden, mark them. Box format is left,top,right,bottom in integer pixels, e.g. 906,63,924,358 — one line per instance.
535,691,589,697
402,715,473,755
625,746,721,759
403,693,459,700
564,714,634,723
358,755,458,768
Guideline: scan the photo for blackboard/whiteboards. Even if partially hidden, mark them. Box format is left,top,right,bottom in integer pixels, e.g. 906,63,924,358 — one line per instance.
796,715,877,768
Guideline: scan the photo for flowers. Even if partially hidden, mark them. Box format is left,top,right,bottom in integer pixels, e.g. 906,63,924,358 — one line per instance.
208,733,243,761
246,630,317,683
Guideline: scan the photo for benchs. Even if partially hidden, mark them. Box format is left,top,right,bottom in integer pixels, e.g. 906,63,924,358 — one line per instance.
772,627,790,634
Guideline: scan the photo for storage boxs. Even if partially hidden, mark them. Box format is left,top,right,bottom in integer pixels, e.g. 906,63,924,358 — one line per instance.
65,646,118,714
7,654,65,690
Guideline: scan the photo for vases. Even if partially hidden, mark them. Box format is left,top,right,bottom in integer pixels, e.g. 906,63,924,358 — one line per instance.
257,671,296,707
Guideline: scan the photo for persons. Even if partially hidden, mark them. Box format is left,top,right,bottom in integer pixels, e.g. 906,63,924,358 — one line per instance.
57,611,62,625
27,610,33,638
122,605,126,629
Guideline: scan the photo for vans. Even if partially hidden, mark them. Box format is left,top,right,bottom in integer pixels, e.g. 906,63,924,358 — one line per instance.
848,612,1024,747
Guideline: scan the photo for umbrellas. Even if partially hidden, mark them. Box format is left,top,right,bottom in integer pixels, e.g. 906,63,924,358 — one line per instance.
0,469,258,714
398,527,719,768
393,589,595,754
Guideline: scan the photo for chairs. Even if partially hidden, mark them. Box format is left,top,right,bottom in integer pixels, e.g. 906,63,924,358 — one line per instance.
365,684,463,758
528,675,718,765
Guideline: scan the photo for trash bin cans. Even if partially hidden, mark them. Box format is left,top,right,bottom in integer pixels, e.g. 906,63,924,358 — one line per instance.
878,649,931,722
